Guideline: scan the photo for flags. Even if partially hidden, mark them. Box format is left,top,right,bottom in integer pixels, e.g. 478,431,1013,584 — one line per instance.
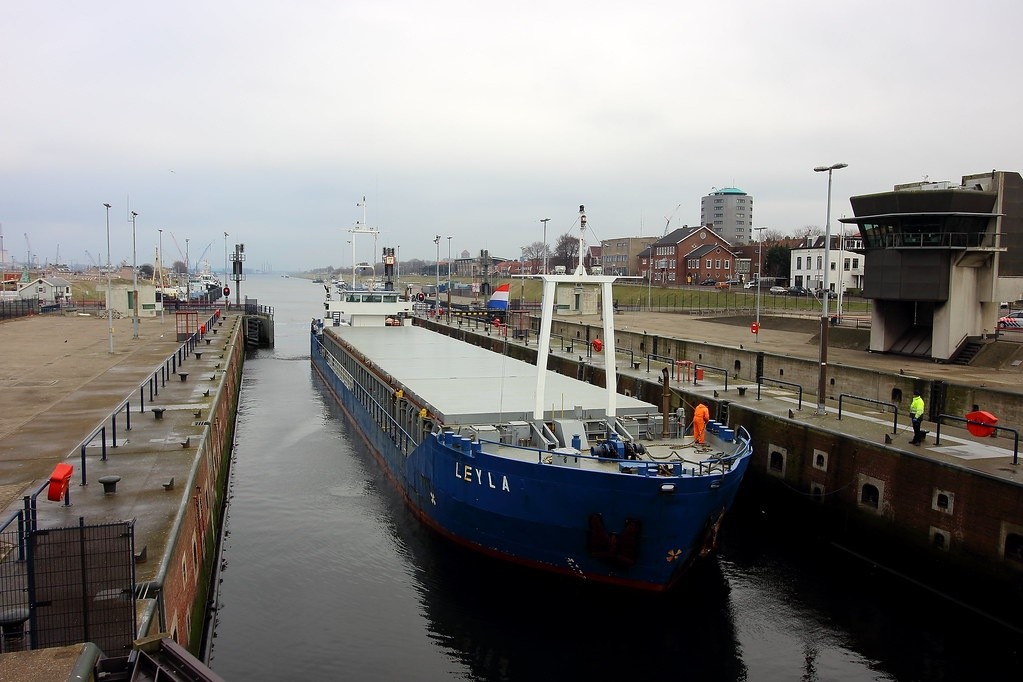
487,282,510,310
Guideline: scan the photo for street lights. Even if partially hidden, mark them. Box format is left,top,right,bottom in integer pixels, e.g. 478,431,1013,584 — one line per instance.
158,229,164,324
224,232,229,312
433,234,442,320
131,211,139,338
447,236,453,323
519,246,526,309
813,161,849,415
540,218,551,274
185,238,190,306
752,227,767,343
397,246,401,292
104,203,113,353
647,247,652,312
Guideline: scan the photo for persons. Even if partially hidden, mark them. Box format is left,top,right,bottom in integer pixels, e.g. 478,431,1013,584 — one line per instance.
908,390,924,446
693,400,709,445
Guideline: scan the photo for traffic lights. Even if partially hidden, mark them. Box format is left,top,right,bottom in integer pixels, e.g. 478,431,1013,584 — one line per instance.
224,288,229,296
419,293,424,301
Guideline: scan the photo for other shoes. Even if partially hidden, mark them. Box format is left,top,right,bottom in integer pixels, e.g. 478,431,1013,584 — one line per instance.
916,442,920,446
909,441,914,443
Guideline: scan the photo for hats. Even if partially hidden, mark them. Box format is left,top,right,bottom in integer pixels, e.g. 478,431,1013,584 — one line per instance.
912,391,920,395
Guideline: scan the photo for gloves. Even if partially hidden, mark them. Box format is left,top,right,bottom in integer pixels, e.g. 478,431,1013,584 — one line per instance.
913,418,918,423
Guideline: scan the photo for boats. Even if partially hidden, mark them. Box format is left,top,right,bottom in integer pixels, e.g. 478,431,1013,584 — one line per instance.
310,204,754,599
312,272,350,290
155,259,221,305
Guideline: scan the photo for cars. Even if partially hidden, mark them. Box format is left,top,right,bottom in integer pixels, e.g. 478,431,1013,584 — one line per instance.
744,281,758,290
998,310,1023,329
788,285,807,296
725,277,741,285
715,281,729,289
770,286,788,296
699,278,717,286
807,287,837,299
